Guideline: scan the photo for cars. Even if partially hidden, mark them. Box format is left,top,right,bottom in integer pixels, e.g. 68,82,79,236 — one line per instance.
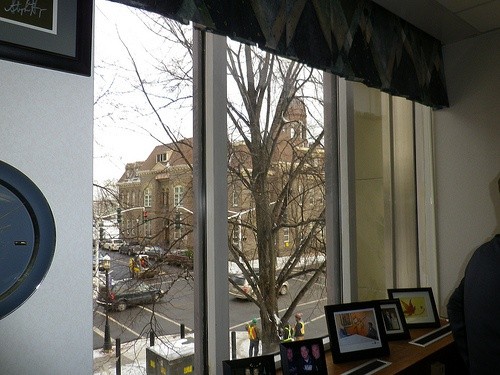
102,238,125,252
228,272,289,302
93,238,109,248
96,278,164,312
118,242,144,256
167,248,193,269
137,246,166,262
93,250,105,271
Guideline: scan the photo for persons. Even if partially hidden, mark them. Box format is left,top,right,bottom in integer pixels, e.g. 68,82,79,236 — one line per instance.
282,321,293,342
245,318,262,358
293,312,305,341
286,345,321,371
447,232,500,375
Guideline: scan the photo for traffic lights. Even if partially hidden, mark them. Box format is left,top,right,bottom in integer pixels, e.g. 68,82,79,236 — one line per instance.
116,208,122,223
233,220,241,245
174,211,181,230
143,211,148,222
283,228,289,244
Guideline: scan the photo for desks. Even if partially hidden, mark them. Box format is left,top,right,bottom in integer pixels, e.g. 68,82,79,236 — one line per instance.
276,318,455,375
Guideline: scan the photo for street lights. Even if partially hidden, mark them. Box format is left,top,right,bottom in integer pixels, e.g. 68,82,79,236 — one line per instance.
101,254,114,353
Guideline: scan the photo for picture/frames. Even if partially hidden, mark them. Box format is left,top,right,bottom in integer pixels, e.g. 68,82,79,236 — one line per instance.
0,0,93,77
372,299,412,340
388,287,439,328
325,300,391,365
279,336,328,375
222,354,276,375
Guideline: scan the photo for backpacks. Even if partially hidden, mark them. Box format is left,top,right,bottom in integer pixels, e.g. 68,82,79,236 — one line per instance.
248,326,257,340
